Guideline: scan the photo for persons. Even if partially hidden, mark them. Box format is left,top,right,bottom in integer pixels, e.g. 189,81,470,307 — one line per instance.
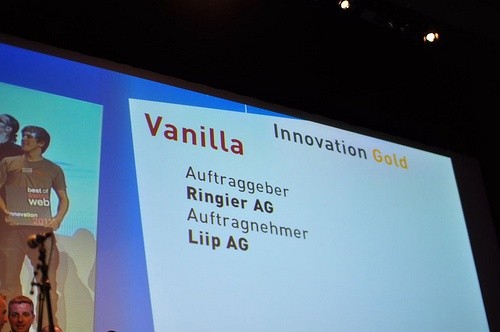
0,125,70,332
0,113,24,161
8,296,35,332
0,293,8,332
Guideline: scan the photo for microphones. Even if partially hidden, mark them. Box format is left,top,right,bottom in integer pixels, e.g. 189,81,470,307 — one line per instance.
27,231,53,249
